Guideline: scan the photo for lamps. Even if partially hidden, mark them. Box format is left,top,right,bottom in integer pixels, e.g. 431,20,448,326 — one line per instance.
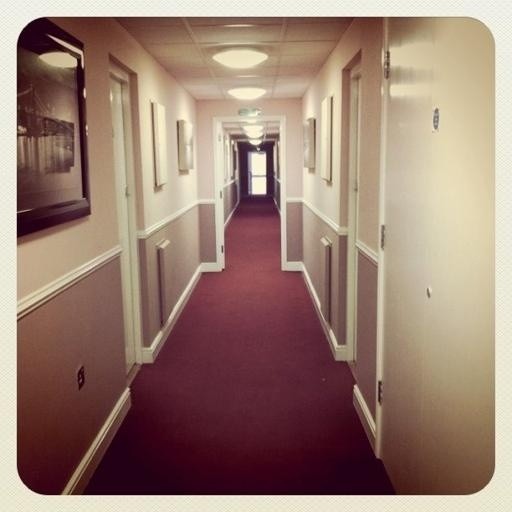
211,45,268,146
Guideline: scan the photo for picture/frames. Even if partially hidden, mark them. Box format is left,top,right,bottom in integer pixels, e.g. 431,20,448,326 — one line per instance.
320,96,332,182
302,118,315,168
17,18,91,237
178,119,193,171
153,101,168,187
